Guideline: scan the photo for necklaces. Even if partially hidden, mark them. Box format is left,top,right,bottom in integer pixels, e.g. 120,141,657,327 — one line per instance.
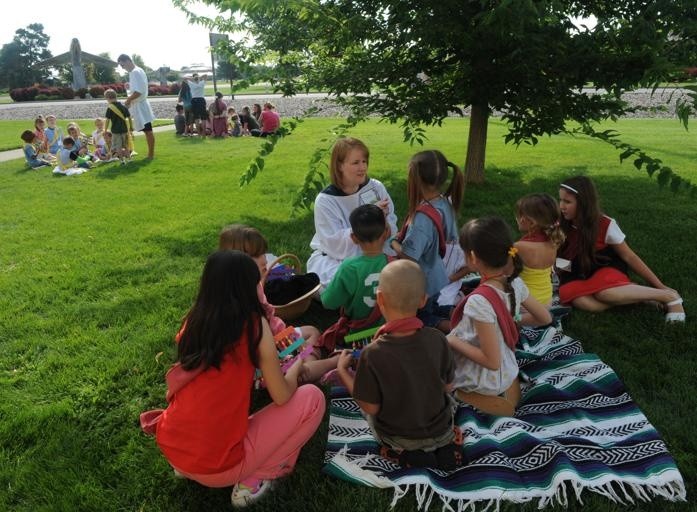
480,270,506,283
422,193,442,202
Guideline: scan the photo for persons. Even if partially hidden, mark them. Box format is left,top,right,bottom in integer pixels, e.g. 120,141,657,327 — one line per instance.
67,122,87,141
389,150,465,335
251,102,280,138
101,128,136,156
137,247,327,508
174,104,186,136
56,136,91,171
104,89,135,168
68,126,83,151
231,114,243,137
251,103,261,120
209,91,227,137
21,129,55,168
219,225,354,391
305,137,398,302
227,107,236,129
444,215,553,417
44,116,64,155
117,54,155,161
337,258,457,470
183,73,207,138
34,115,49,153
512,191,566,314
178,78,194,137
555,175,685,329
92,118,109,160
320,203,397,344
239,106,260,131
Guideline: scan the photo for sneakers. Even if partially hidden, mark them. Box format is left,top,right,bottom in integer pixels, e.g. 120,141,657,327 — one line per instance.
230,481,277,510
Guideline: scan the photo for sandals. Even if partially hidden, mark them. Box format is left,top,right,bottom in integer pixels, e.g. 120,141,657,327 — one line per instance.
666,295,686,325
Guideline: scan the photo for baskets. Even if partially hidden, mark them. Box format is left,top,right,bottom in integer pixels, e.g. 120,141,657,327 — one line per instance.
260,252,321,322
